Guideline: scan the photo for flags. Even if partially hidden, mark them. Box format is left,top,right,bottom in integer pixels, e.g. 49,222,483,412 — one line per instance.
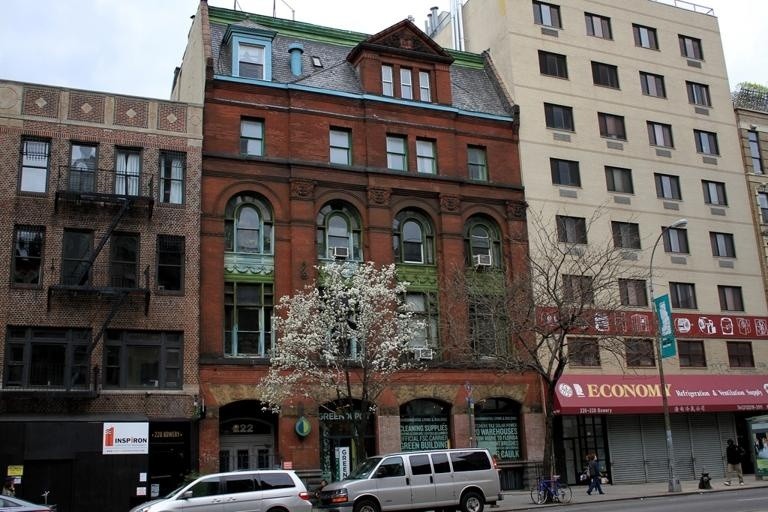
652,293,676,360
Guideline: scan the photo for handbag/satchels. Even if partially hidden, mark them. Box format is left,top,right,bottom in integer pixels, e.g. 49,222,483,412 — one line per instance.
601,478,608,484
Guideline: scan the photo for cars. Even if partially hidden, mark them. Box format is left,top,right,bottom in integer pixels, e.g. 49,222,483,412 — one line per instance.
0,494,59,512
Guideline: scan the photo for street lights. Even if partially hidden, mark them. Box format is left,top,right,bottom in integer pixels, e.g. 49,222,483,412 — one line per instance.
648,218,689,494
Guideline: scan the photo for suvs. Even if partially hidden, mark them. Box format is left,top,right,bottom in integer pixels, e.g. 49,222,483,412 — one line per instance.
319,446,506,512
129,467,315,511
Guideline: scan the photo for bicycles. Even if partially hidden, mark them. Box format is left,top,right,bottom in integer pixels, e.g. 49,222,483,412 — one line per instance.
530,474,573,504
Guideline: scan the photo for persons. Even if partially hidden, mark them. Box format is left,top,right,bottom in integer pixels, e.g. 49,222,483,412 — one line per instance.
2,481,16,499
313,478,328,498
720,438,745,486
489,454,501,508
584,455,603,492
585,453,606,495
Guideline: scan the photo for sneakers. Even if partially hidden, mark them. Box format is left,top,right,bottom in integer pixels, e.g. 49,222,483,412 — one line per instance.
725,481,744,485
587,491,605,495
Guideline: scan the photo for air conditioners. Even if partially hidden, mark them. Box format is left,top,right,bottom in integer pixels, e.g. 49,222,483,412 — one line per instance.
334,247,348,260
415,348,432,360
473,254,491,271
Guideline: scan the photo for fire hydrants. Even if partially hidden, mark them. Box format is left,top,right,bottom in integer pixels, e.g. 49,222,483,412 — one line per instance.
700,471,712,488
